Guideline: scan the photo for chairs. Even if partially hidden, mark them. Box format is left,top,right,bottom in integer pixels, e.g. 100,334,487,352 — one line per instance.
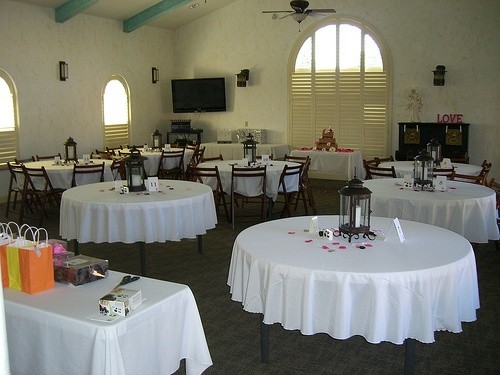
411,160,492,187
7,139,316,230
361,155,397,180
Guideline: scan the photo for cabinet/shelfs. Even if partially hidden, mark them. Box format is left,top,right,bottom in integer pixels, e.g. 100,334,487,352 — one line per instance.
395,122,471,164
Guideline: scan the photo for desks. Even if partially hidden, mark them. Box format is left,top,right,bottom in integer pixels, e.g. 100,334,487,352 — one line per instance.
58,180,218,277
200,142,289,160
227,215,480,375
289,148,365,181
197,160,302,222
346,178,500,243
2,269,213,375
105,147,194,180
15,159,122,191
374,161,483,182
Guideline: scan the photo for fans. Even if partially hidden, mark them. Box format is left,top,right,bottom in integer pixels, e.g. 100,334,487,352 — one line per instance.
262,0,337,20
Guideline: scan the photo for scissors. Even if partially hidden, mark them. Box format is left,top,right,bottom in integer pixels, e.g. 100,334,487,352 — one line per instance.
111,276,140,293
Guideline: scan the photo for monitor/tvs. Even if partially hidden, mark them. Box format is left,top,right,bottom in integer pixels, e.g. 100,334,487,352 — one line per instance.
171,77,227,113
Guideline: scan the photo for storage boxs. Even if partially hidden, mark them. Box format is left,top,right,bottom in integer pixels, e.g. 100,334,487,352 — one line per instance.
99,288,141,317
39,235,108,286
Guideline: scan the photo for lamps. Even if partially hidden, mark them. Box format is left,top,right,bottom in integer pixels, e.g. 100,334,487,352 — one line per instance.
432,65,448,86
151,67,159,83
290,12,308,32
59,61,68,81
235,69,249,87
